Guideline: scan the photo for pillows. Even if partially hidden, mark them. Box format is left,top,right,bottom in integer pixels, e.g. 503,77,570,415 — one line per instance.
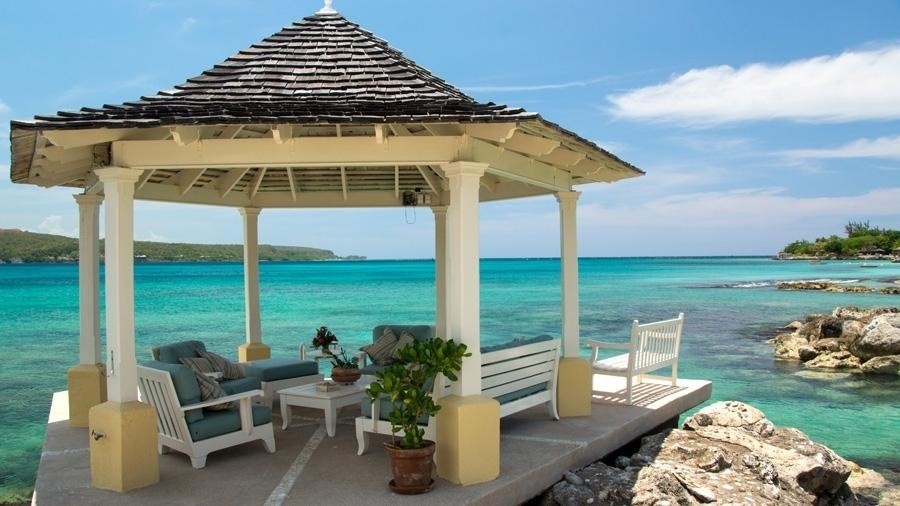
359,328,418,366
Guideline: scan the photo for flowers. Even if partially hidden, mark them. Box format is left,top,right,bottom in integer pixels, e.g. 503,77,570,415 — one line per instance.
312,326,358,368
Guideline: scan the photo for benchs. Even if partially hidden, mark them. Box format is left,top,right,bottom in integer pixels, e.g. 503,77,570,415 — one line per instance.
584,313,685,405
354,332,563,457
243,356,323,411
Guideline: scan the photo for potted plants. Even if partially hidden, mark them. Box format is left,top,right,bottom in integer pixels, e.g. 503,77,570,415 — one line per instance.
365,336,473,495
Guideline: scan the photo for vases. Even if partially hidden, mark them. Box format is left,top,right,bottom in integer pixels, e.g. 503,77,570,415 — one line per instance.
332,364,360,382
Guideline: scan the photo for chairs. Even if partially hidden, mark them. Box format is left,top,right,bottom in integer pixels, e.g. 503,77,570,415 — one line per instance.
135,340,278,470
357,326,435,426
